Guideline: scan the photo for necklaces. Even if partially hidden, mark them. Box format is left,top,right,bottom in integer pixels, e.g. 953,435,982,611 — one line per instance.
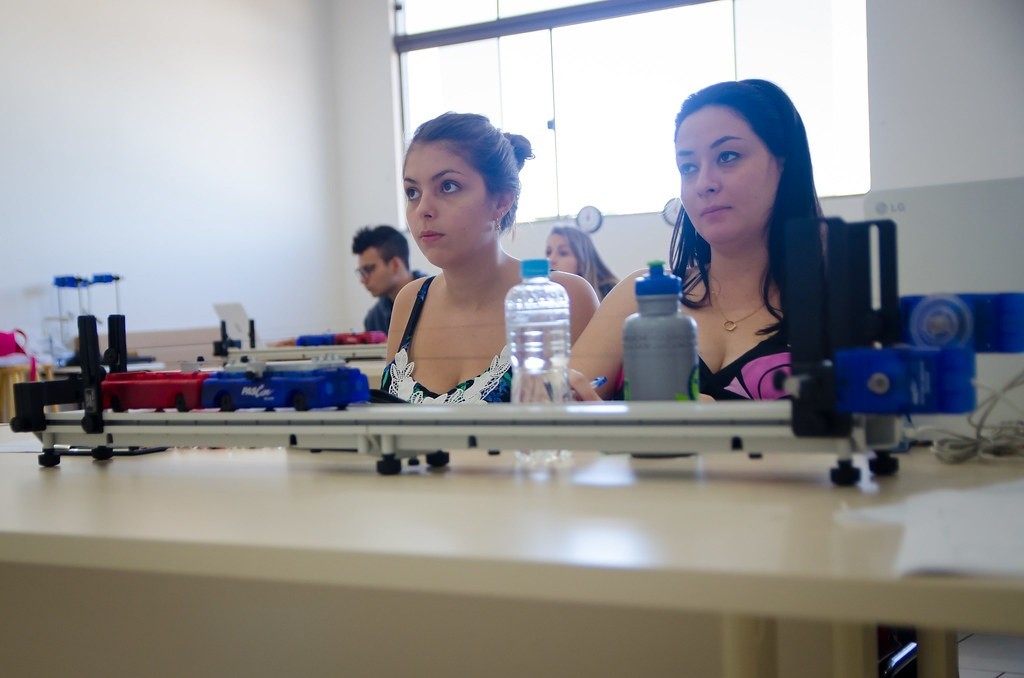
708,270,777,331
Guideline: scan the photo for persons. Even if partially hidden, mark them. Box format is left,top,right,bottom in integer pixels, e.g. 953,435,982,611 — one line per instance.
381,112,601,404
353,225,427,337
518,79,833,404
546,226,619,303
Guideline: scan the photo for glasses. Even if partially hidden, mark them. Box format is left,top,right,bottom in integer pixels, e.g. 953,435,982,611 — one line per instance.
357,259,388,276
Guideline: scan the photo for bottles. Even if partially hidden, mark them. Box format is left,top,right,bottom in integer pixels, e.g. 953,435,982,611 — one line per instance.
503,257,574,463
620,259,702,459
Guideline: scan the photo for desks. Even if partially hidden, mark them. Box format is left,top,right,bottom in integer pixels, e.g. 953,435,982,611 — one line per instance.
0,422,1023,678
